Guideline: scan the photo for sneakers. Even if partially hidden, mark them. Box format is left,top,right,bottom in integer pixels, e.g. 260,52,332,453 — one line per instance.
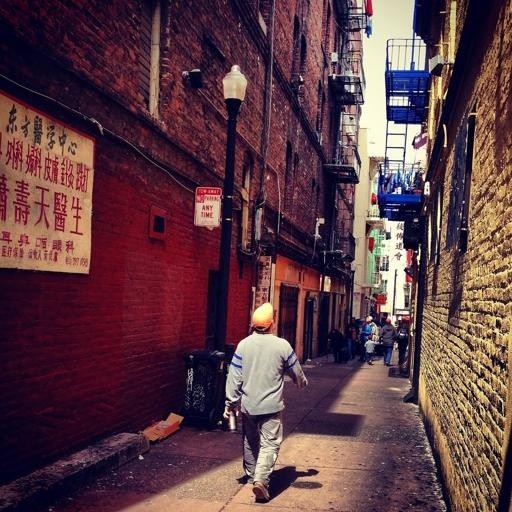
384,361,390,366
363,360,367,362
252,481,270,502
357,359,362,361
246,477,254,484
369,360,372,364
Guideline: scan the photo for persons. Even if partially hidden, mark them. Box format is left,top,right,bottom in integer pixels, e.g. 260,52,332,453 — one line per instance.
223,302,309,503
328,309,410,366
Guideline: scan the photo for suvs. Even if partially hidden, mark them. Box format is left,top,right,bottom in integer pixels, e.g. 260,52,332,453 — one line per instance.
354,318,384,356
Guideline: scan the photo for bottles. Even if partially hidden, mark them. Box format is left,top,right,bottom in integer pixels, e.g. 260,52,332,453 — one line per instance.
227,409,238,435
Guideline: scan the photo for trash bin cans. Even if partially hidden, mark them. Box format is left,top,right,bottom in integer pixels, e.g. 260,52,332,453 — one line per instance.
177,351,226,428
327,331,354,364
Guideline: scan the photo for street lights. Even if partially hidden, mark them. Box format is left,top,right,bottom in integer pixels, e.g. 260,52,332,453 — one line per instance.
210,63,248,353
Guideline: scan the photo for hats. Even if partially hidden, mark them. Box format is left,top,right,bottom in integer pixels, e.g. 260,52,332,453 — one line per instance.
385,318,392,324
250,302,275,333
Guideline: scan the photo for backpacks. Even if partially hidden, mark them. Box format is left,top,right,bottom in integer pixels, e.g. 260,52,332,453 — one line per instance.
397,328,408,340
